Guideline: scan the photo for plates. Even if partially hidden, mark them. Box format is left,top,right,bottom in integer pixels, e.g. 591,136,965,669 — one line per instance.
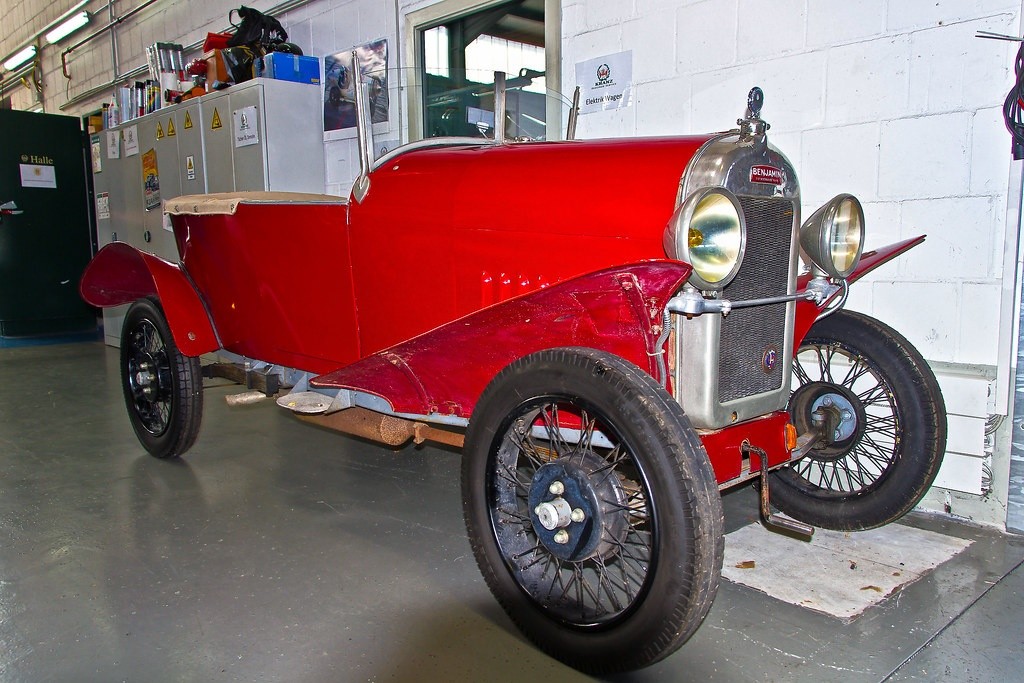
276,392,335,413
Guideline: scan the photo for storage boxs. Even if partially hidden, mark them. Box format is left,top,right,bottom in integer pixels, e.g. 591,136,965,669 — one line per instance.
251,51,320,85
88,116,103,134
202,49,230,92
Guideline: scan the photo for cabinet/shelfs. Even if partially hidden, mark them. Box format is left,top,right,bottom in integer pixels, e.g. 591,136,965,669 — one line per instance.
88,77,326,365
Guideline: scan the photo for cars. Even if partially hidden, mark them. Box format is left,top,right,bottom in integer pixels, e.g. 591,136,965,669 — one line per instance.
82,74,947,674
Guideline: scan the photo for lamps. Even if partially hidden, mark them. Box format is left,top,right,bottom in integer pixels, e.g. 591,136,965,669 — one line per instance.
45,11,93,44
3,44,39,71
